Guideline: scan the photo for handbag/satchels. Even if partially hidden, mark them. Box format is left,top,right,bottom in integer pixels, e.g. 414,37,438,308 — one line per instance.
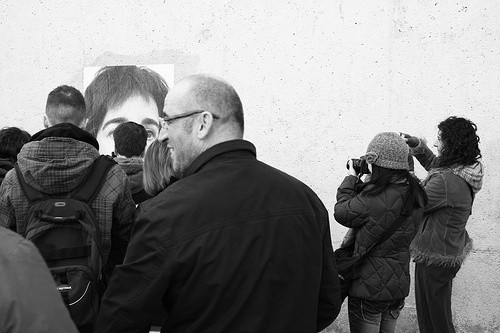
333,239,362,280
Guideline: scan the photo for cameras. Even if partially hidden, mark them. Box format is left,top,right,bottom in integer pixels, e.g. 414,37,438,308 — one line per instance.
347,158,371,176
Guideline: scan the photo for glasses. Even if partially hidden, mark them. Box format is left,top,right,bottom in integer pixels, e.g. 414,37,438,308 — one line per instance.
164,110,220,125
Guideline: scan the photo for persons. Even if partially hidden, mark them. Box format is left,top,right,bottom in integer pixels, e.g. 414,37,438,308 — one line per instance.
93,76,342,333
144,138,174,198
399,116,483,333
334,132,428,333
1,226,80,333
112,122,153,204
86,67,170,155
1,85,136,333
1,127,32,182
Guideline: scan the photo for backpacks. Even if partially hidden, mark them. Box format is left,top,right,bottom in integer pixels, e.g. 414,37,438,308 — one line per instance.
14,155,119,333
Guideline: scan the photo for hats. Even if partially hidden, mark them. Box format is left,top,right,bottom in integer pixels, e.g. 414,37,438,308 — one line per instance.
360,133,414,171
